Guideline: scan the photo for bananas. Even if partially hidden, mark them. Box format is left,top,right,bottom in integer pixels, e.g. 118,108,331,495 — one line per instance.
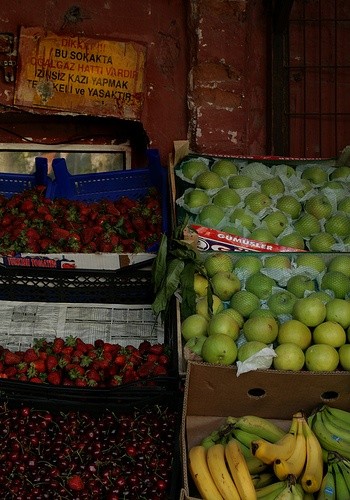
187,402,350,500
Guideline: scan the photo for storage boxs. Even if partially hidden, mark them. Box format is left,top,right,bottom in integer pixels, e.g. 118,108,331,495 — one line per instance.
168,149,344,253
3,390,182,500
0,157,53,264
180,361,350,500
1,264,183,395
172,264,315,376
51,150,170,270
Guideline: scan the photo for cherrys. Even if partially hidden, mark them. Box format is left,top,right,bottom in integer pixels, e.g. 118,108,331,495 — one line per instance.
0,400,184,500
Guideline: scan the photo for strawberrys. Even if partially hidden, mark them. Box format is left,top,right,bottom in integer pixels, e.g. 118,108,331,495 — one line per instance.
0,330,170,389
0,184,163,260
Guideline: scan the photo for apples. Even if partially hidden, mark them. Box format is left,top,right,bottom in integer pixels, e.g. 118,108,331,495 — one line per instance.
177,158,350,252
181,252,350,371
